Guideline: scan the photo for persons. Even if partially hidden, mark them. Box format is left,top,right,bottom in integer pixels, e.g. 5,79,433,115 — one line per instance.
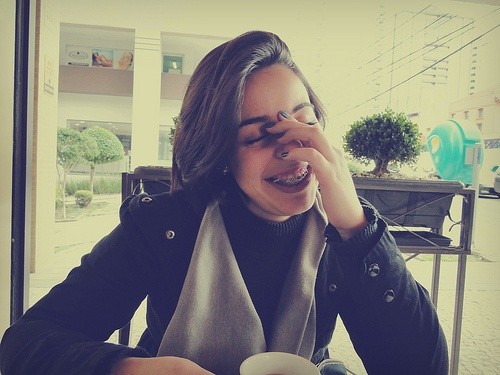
91,51,112,66
0,30,450,375
118,52,133,69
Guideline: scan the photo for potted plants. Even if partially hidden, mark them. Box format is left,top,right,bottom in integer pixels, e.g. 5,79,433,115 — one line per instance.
343,108,465,229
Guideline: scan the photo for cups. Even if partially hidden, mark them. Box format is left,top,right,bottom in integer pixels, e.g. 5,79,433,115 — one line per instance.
240,351,320,375
